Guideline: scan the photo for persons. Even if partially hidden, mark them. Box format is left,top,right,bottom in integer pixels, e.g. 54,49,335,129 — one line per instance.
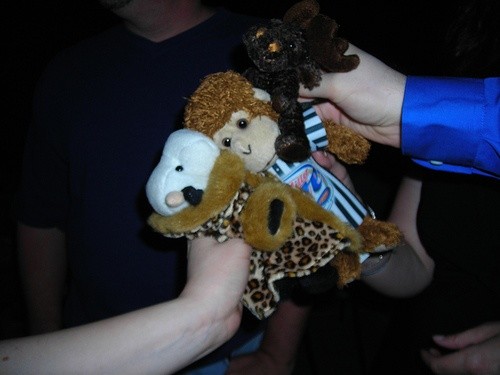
314,0,500,375
12,0,315,375
0,232,253,375
297,40,500,180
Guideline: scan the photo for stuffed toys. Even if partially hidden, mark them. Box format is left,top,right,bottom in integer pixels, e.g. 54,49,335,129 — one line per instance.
145,128,360,322
182,70,401,287
239,8,359,163
